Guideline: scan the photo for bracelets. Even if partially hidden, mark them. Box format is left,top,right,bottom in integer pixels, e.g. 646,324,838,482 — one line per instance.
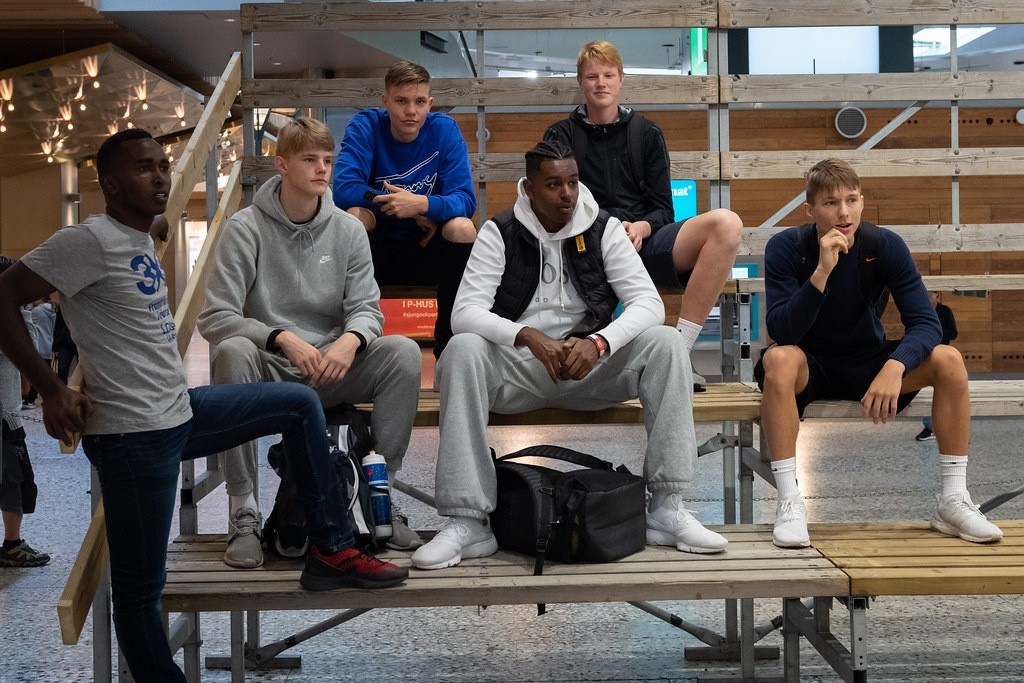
587,334,604,358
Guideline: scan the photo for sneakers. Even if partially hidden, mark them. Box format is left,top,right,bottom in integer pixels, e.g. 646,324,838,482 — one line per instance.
0,538,50,567
411,515,497,568
930,489,1003,542
772,490,811,548
645,493,728,552
224,507,263,567
916,427,936,440
300,544,409,590
378,503,422,549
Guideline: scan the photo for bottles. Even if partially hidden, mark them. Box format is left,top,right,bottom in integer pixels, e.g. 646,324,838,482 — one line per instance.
361,450,393,537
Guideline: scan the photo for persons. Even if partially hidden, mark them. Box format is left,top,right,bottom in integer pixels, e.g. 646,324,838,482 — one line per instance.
0,129,409,683
332,61,477,356
904,292,958,441
754,159,1003,548
196,117,423,568
543,40,742,390
412,140,728,571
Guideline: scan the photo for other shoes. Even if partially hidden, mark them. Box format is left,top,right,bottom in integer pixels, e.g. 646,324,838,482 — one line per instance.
22,401,35,408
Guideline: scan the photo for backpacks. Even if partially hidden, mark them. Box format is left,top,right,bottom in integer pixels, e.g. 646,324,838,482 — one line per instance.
263,374,376,557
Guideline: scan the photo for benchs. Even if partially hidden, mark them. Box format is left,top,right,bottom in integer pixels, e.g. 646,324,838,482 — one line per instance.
53,272,1024,683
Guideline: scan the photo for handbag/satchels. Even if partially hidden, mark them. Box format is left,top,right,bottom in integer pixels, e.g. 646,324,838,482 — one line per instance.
487,444,647,615
